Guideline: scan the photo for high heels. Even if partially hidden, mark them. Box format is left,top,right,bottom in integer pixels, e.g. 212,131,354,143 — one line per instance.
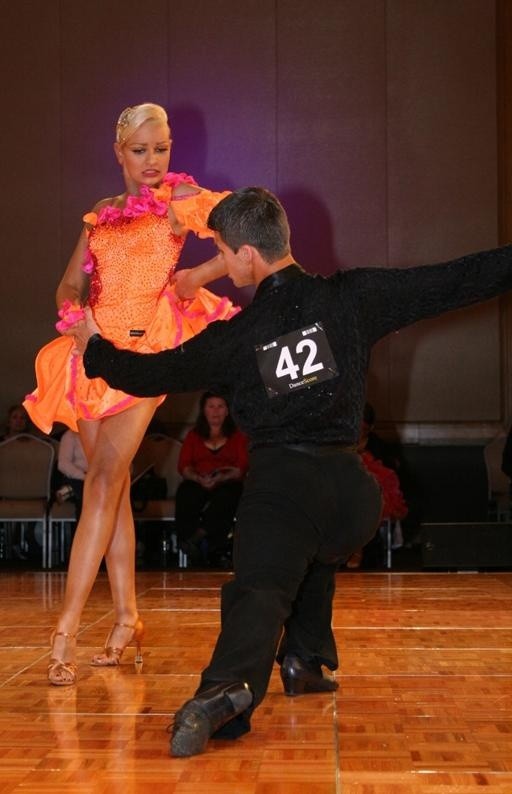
47,625,81,685
89,615,147,667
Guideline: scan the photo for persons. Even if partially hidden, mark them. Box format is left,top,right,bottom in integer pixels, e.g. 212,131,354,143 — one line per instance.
172,388,248,572
346,401,425,572
18,100,244,687
59,185,511,759
55,428,87,508
1,404,30,440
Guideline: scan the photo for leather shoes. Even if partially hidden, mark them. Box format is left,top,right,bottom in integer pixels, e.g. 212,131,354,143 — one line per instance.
168,677,255,759
279,652,340,696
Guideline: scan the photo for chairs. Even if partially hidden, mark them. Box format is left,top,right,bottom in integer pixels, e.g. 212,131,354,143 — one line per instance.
0,431,189,570
